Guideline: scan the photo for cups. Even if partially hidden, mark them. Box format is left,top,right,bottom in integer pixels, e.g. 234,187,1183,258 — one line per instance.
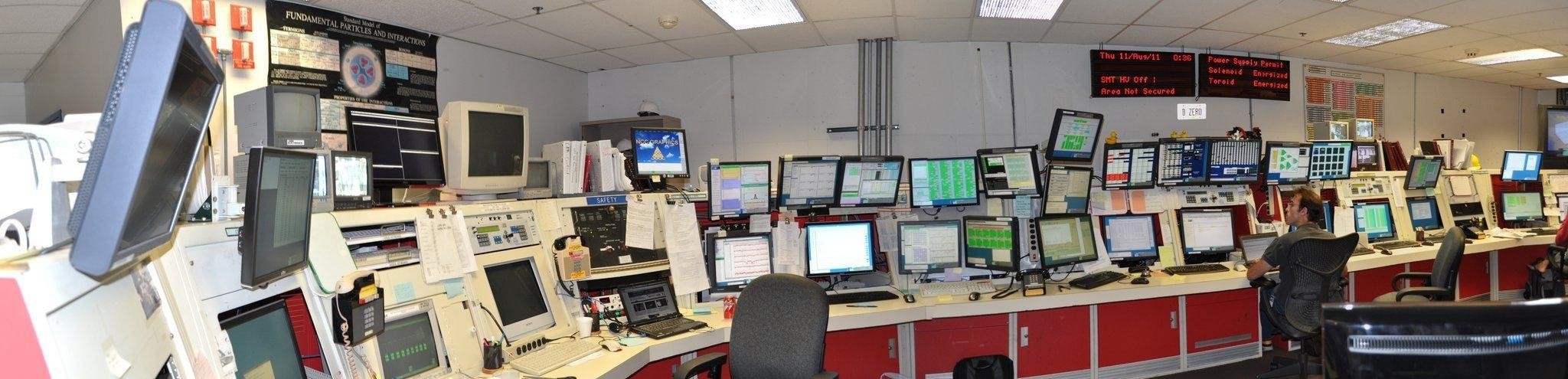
577,316,594,339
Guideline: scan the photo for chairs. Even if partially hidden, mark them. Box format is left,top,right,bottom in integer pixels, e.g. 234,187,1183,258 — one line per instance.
1258,234,1364,377
1374,223,1466,304
1531,242,1568,298
677,274,838,377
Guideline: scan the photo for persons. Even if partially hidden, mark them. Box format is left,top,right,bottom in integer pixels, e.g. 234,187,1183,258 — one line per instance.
1225,126,1261,141
1169,130,1178,138
1102,130,1118,145
1245,188,1349,352
1526,208,1568,297
1180,129,1187,139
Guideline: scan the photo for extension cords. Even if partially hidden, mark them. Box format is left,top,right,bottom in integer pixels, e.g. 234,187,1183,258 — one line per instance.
1266,185,1275,217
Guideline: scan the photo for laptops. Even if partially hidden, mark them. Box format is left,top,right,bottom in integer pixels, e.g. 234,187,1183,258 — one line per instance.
617,279,709,340
1239,232,1280,272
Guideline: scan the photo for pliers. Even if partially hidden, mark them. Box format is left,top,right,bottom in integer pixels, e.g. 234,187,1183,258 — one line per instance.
1057,284,1071,292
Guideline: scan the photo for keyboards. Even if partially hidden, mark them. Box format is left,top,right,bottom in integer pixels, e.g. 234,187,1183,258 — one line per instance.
1069,270,1127,290
919,279,996,298
509,339,603,376
822,291,899,304
1163,263,1230,275
1351,248,1376,256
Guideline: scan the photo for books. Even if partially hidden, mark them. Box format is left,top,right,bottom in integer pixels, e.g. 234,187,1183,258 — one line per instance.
1381,138,1475,171
542,139,633,195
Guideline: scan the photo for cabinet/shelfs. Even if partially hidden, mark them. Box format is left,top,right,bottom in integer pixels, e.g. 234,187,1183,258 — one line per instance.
1408,258,1435,288
1460,252,1489,300
1497,244,1547,291
1352,263,1405,304
909,313,1014,379
1183,288,1261,372
1014,304,1093,379
825,323,906,379
1095,295,1184,379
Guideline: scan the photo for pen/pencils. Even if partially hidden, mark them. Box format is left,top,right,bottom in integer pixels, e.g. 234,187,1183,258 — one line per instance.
1415,226,1424,241
482,332,506,369
583,301,599,331
619,343,627,346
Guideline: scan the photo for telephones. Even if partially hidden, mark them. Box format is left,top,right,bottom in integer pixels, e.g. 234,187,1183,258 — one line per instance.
1015,268,1051,297
553,233,591,282
331,268,386,348
1462,223,1485,239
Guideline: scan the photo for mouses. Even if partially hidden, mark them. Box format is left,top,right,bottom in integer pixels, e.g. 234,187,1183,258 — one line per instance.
1381,249,1392,255
599,339,622,352
1233,265,1247,272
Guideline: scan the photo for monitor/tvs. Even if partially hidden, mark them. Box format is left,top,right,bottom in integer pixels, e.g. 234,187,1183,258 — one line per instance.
1098,141,1160,267
1034,108,1104,267
1313,121,1349,140
65,0,557,379
1350,107,1568,250
1288,200,1334,234
1320,296,1568,379
1156,136,1210,187
1179,208,1236,255
897,145,1042,274
630,127,691,194
1264,141,1314,185
706,154,905,292
1306,140,1354,182
1208,136,1262,185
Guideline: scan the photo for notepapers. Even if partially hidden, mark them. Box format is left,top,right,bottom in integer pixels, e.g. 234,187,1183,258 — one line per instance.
693,308,713,315
